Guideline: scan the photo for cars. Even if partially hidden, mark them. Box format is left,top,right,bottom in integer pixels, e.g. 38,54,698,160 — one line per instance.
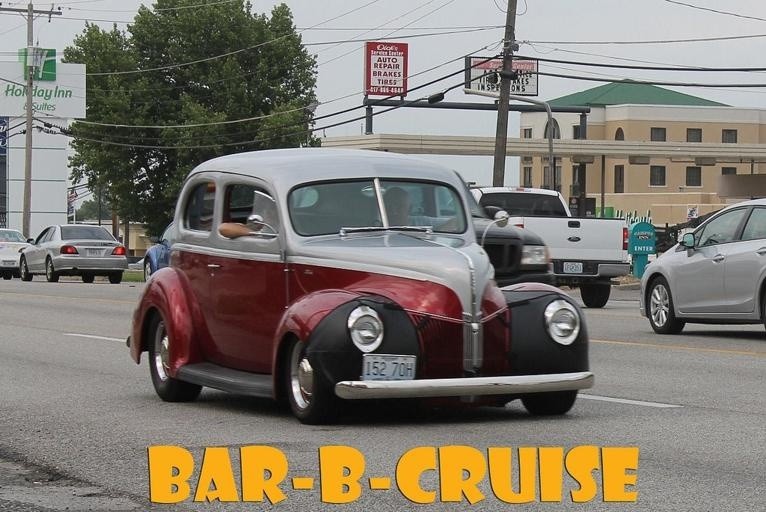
0,224,128,283
640,199,766,334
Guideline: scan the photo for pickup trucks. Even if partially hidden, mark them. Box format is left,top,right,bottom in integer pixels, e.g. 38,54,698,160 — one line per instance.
469,186,634,308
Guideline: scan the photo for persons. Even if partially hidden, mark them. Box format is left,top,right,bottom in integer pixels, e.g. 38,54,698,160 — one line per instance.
217,185,307,237
381,186,450,228
